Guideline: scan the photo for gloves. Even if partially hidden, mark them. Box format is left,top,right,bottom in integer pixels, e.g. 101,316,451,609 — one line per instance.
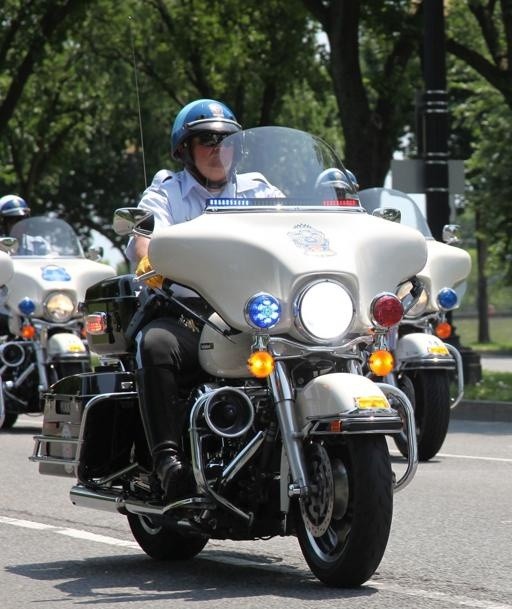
134,255,164,290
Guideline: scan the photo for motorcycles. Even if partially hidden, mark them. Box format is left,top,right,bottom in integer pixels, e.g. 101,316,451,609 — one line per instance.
27,125,431,588
0,216,118,430
355,187,474,462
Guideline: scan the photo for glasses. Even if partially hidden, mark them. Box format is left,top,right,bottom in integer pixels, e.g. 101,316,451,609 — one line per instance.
190,132,234,147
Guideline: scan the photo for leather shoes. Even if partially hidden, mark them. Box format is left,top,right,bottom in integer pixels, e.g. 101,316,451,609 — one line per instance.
156,453,189,503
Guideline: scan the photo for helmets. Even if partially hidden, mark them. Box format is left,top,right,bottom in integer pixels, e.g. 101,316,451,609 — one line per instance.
170,99,243,162
0,195,31,221
314,168,360,194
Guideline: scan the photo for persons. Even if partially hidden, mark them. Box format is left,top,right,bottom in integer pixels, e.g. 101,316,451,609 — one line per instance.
313,167,360,204
3,194,101,342
121,96,287,507
